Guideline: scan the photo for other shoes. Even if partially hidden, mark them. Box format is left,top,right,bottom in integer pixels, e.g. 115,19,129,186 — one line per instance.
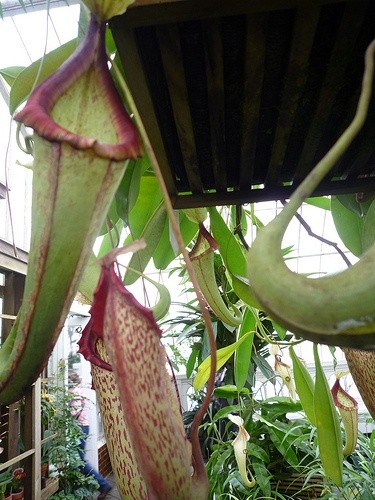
97,486,112,499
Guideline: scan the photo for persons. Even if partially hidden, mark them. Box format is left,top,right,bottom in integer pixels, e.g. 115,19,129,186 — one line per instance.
185,371,206,411
66,371,115,500
